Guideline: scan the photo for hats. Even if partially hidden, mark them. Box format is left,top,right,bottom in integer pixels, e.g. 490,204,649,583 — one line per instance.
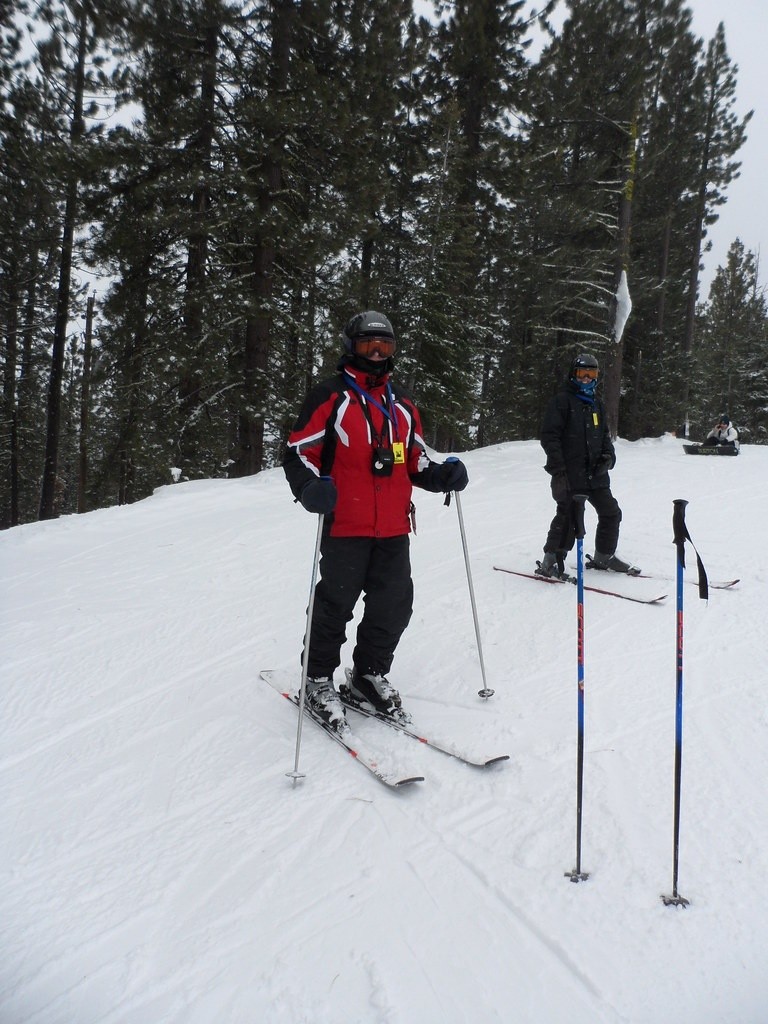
720,416,729,425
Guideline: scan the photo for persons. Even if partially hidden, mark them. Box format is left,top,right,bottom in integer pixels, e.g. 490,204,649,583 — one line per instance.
281,310,470,727
701,416,740,451
534,353,640,586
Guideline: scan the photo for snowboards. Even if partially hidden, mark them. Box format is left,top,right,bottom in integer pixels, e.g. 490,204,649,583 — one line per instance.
683,444,739,457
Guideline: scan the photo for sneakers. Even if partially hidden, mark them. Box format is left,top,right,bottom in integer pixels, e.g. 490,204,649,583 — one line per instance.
340,663,412,726
585,549,640,576
299,676,353,741
534,551,578,584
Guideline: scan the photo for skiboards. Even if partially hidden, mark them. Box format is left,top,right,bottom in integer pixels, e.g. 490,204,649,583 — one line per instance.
259,667,512,791
491,553,742,604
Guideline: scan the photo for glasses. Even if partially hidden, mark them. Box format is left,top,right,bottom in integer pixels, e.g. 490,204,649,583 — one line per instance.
574,367,598,378
352,334,395,357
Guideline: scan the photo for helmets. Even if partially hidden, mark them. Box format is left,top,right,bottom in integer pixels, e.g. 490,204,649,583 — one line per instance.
570,353,599,379
342,311,395,352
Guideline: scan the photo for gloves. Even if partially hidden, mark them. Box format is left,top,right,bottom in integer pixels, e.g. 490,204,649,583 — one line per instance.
434,457,469,491
550,472,571,502
721,439,728,444
301,478,338,514
592,454,612,477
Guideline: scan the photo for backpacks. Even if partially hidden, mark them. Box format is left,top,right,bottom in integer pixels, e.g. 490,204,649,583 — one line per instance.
718,426,740,440
